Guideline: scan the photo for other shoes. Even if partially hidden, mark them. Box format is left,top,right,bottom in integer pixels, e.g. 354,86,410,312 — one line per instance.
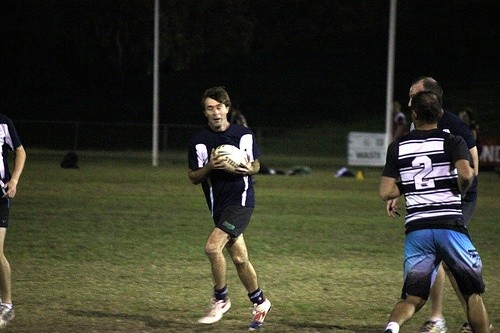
419,318,448,333
460,322,492,333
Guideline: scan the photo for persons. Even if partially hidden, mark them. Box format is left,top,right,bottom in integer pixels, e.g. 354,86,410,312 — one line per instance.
393,101,406,141
188,87,273,332
385,76,479,333
377,90,492,333
458,106,483,157
0,113,27,329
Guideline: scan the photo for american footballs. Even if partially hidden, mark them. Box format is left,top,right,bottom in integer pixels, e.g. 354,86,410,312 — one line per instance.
214,144,249,175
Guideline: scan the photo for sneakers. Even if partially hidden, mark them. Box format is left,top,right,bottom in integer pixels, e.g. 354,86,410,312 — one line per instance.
249,294,272,331
198,296,231,324
0,299,15,328
